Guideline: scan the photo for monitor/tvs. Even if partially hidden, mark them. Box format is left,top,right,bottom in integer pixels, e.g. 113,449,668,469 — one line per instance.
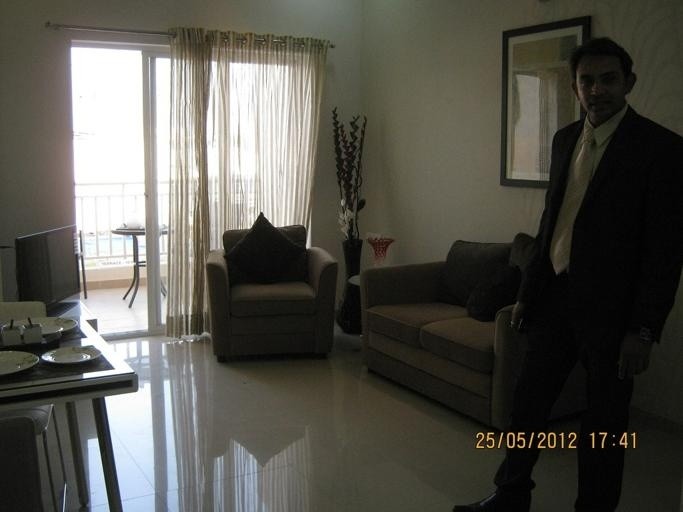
14,225,81,318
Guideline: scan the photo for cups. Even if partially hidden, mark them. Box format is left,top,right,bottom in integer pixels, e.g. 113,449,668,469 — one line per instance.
0,324,41,346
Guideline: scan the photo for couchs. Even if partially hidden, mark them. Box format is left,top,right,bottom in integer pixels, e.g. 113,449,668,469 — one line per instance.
359,260,534,431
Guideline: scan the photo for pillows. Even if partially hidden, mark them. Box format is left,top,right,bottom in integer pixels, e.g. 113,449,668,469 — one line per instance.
508,230,535,266
435,239,513,304
221,212,307,284
466,264,521,321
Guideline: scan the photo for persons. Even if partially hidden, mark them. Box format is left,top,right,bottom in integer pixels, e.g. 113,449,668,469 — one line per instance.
452,38,683,512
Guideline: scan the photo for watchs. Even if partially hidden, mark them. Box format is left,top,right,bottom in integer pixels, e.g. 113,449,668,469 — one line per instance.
628,325,655,344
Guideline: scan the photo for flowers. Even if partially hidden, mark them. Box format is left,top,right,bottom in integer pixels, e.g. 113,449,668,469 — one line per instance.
331,104,367,237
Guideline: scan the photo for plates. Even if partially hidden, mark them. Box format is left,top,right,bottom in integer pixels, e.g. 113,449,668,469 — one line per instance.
9,317,77,335
0,351,39,374
41,346,100,365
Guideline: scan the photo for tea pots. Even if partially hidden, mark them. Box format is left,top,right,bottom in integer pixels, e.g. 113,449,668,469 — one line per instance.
126,222,144,229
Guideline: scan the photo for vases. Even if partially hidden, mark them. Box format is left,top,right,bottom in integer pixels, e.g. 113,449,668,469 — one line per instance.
368,237,394,265
336,239,363,335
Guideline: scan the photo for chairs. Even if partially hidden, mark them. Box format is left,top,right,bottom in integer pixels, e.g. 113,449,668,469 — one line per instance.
0,301,68,512
206,223,338,361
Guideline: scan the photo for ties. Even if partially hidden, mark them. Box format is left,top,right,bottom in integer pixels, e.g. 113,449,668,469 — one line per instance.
549,130,595,275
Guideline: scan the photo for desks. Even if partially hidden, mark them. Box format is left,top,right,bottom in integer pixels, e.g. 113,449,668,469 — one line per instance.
112,227,175,309
47,299,98,332
348,275,360,287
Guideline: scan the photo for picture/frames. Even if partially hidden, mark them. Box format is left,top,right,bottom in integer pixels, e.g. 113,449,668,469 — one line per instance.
500,15,592,187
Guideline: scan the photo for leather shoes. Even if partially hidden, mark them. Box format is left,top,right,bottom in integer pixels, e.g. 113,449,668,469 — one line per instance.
452,487,531,512
